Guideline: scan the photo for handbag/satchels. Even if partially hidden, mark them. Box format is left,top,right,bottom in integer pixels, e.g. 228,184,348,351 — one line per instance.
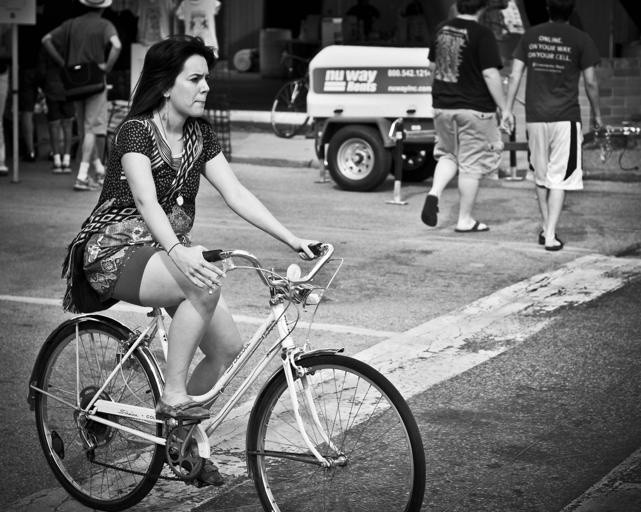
61,27,106,100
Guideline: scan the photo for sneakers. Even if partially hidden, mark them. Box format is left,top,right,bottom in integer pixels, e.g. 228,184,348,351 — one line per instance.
52,164,71,174
73,176,100,191
95,172,106,184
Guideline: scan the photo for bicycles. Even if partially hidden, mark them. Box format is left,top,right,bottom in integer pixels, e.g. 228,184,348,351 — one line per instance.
271,52,313,138
26,242,427,511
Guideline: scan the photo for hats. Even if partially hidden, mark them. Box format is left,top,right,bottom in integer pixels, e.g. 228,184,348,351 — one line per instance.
80,1,113,8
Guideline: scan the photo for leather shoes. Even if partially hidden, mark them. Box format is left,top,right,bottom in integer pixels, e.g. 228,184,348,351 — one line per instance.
153,395,209,421
169,449,225,487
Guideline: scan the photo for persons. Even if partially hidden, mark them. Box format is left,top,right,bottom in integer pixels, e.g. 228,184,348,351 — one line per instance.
417,0,506,233
0,0,121,190
59,41,323,485
499,0,603,249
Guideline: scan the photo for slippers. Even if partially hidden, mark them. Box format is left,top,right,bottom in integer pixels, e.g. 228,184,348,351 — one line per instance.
454,219,489,232
545,237,563,251
539,228,558,244
420,194,438,227
